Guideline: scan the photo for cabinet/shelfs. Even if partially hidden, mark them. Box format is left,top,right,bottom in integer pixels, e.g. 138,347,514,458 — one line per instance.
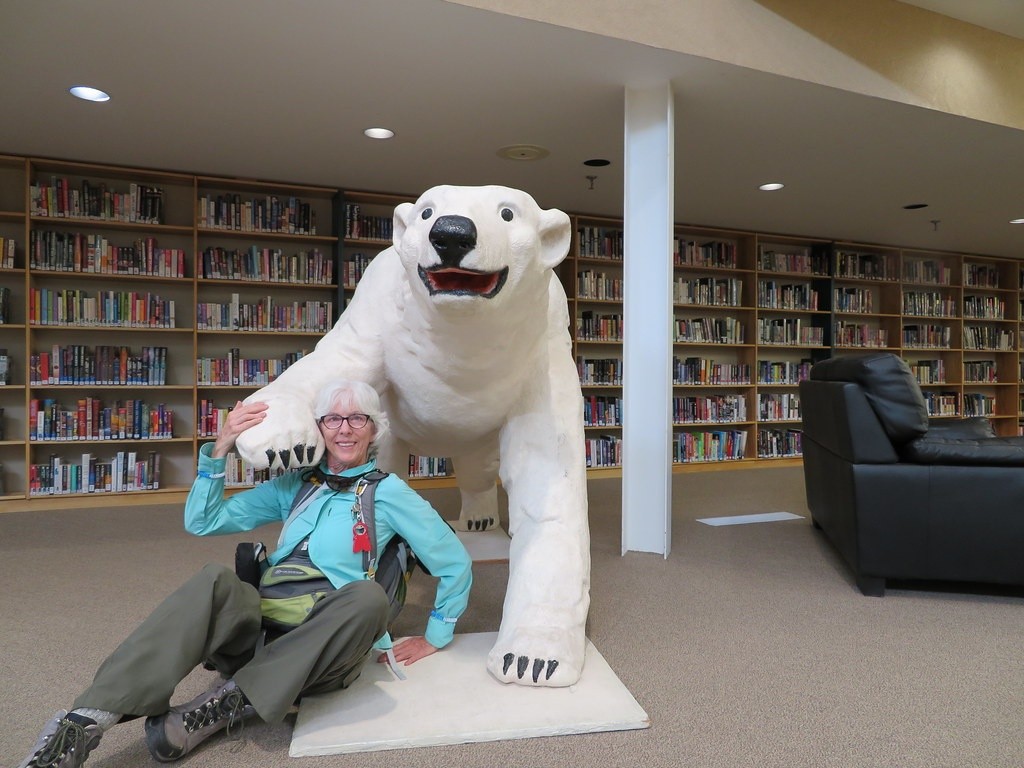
0,155,342,513
341,190,833,492
833,242,1024,437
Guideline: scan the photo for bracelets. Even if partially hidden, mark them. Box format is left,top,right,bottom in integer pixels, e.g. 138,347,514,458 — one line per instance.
198,469,227,480
430,611,458,625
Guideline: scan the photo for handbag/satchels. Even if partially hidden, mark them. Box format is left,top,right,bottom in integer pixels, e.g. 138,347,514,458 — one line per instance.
258,555,336,632
235,541,269,590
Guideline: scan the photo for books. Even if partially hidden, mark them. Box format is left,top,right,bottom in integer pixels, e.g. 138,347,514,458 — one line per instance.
672,226,755,462
555,217,624,470
1017,260,1024,439
901,258,961,418
344,199,407,314
407,452,448,478
0,236,17,423
757,239,828,459
27,171,193,497
962,260,1016,440
831,249,899,349
196,191,339,489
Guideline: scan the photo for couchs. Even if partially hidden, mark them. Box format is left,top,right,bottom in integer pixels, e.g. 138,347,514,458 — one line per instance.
799,353,1024,597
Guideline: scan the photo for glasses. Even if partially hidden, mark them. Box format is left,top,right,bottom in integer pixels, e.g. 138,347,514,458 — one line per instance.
319,413,372,430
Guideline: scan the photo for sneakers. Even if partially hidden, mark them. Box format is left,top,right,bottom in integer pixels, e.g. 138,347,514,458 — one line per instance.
145,680,258,763
18,709,103,768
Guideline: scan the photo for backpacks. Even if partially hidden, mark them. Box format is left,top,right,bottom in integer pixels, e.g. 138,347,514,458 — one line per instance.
287,470,417,629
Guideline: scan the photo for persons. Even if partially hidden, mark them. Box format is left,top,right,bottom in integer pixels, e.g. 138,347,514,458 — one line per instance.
24,378,473,768
